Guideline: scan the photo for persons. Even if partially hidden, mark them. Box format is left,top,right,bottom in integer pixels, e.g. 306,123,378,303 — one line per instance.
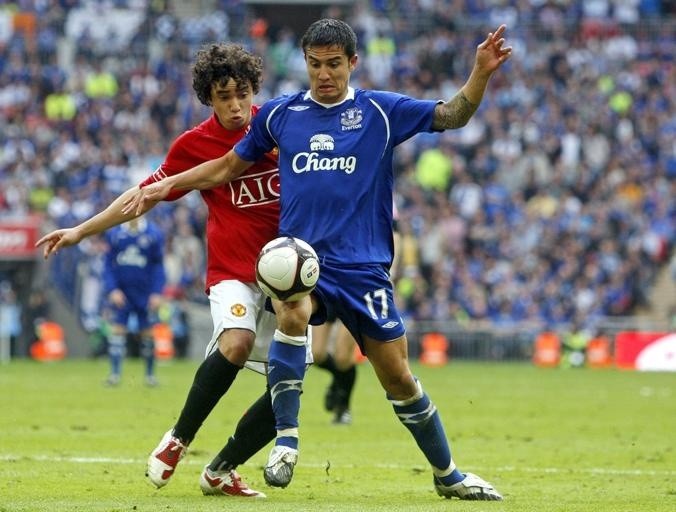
311,318,357,426
120,18,513,502
1,1,675,390
33,40,316,498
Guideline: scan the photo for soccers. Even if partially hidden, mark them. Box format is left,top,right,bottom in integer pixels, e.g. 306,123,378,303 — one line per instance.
255,237,319,302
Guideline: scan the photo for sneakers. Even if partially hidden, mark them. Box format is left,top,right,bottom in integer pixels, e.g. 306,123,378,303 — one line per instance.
264,443,299,488
433,471,503,501
325,379,336,412
331,412,350,426
197,465,267,499
99,372,161,389
146,427,188,488
